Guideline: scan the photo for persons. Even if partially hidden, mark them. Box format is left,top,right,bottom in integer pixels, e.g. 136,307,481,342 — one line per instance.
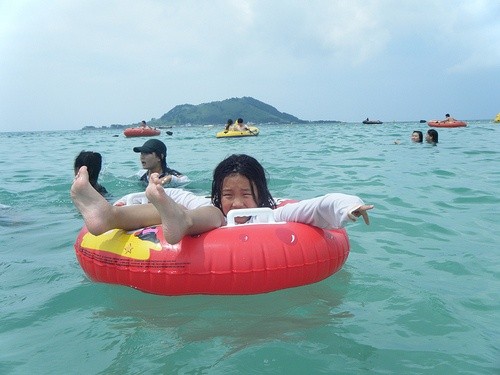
71,118,374,244
412,130,423,143
439,114,457,123
425,129,438,144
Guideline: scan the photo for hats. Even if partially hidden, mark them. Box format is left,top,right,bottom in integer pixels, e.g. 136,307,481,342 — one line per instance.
133,139,167,153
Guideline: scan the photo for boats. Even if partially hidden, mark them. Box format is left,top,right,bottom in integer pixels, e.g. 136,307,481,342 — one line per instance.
362,120,383,124
124,127,161,138
428,120,467,127
216,127,259,138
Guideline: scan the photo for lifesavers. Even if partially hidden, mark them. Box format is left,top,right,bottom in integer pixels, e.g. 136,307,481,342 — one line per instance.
73,197,350,297
